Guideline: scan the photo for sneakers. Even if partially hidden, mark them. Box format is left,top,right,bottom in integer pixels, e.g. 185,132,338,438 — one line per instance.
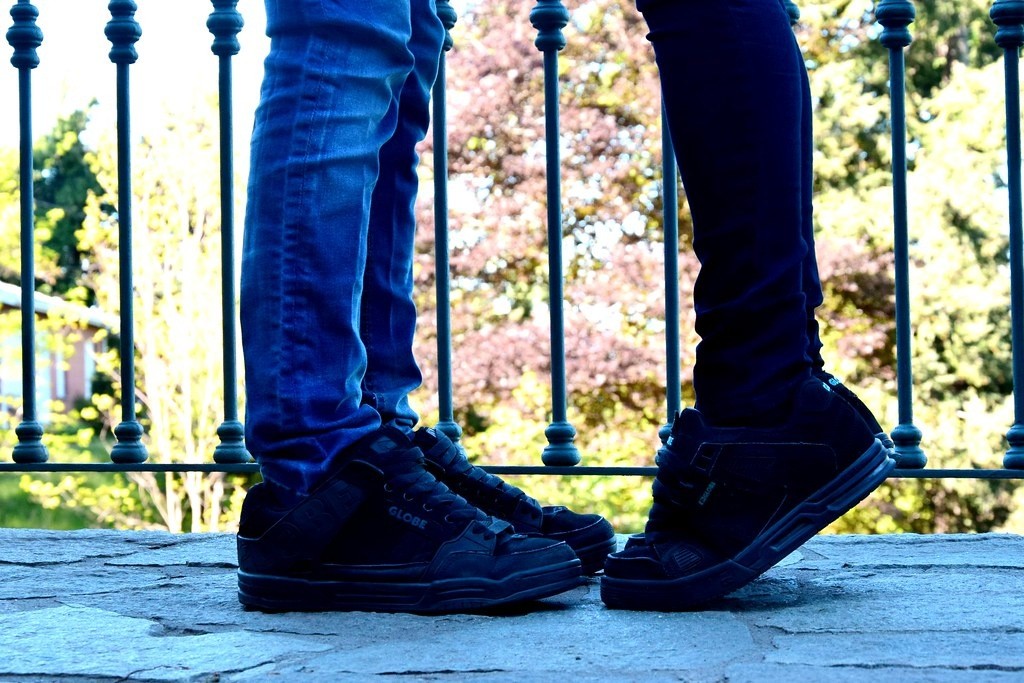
599,365,904,611
236,425,583,614
623,368,893,550
407,427,617,570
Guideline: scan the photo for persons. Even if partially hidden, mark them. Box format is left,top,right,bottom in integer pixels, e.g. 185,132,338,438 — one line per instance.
236,0,618,618
600,0,902,613
